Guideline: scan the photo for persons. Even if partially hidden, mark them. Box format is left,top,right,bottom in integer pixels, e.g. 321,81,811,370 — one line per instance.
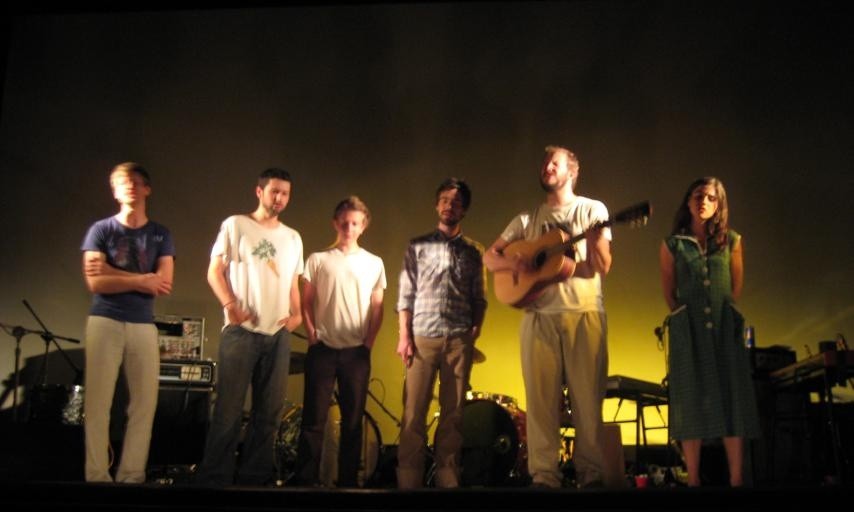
479,144,613,490
656,173,756,488
392,174,489,489
78,158,177,486
293,194,387,488
188,165,307,488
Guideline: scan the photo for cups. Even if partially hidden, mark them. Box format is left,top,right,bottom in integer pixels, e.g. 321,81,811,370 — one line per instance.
634,475,651,488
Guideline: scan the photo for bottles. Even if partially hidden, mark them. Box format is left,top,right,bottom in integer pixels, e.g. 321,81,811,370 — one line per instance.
835,332,850,350
745,324,755,348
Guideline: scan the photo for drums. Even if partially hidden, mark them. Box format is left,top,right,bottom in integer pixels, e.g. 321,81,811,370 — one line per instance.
433,392,527,488
276,400,382,488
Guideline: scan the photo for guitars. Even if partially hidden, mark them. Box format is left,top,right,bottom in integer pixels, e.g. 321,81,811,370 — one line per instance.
493,200,652,309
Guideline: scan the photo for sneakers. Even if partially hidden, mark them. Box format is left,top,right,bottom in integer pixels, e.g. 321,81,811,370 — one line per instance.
393,470,466,491
531,470,609,494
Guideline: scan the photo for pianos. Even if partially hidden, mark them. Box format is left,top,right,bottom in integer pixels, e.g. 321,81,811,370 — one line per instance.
565,375,669,406
